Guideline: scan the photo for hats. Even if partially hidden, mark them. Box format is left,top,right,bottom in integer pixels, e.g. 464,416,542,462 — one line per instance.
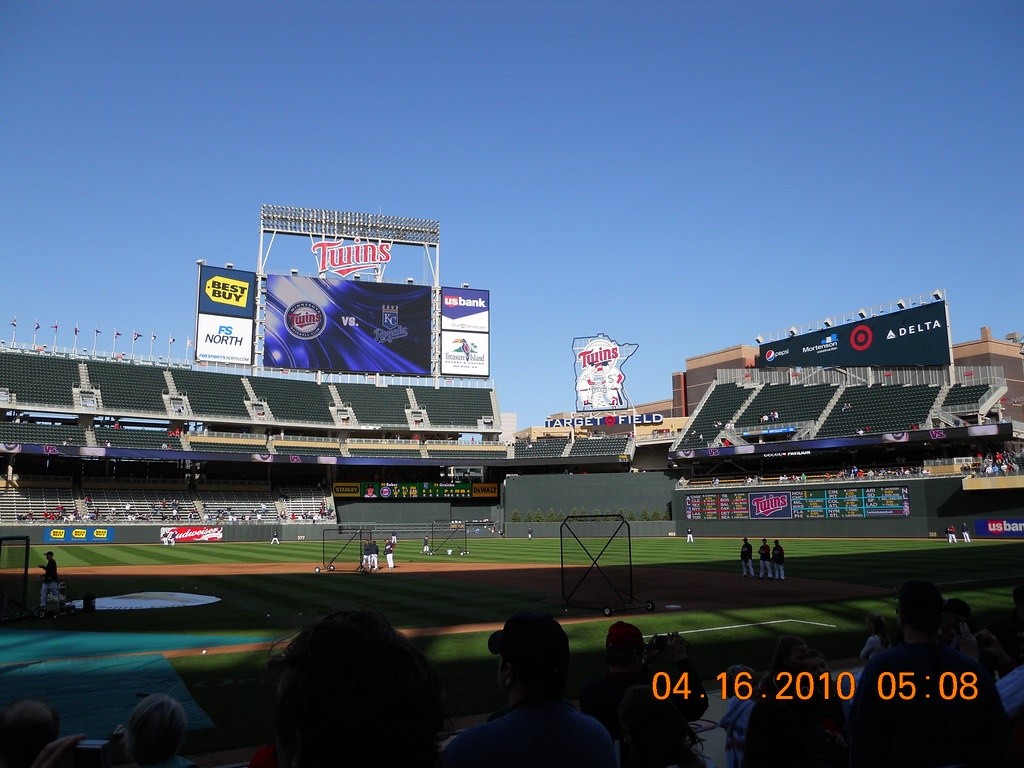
940,598,970,616
43,551,54,556
384,538,388,541
487,611,570,671
606,622,648,650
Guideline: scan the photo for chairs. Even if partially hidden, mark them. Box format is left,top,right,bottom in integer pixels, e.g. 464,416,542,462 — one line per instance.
515,438,637,457
676,384,1023,479
0,352,508,525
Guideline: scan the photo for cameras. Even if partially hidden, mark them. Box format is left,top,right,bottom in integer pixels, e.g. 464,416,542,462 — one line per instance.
56,740,113,768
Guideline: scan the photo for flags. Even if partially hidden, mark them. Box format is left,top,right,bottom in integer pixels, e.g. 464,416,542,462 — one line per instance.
9,320,193,347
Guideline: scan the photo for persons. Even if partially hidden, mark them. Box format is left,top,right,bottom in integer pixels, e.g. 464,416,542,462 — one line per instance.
779,473,806,484
525,436,532,450
744,475,764,485
711,477,719,487
687,527,693,542
740,537,784,580
62,424,180,449
528,527,532,540
363,531,397,571
163,532,176,545
37,551,59,609
854,415,960,435
944,522,970,543
825,466,930,482
842,403,850,411
0,582,1024,768
699,410,778,442
271,532,279,545
960,449,1024,476
18,496,335,524
364,484,377,497
420,535,429,555
